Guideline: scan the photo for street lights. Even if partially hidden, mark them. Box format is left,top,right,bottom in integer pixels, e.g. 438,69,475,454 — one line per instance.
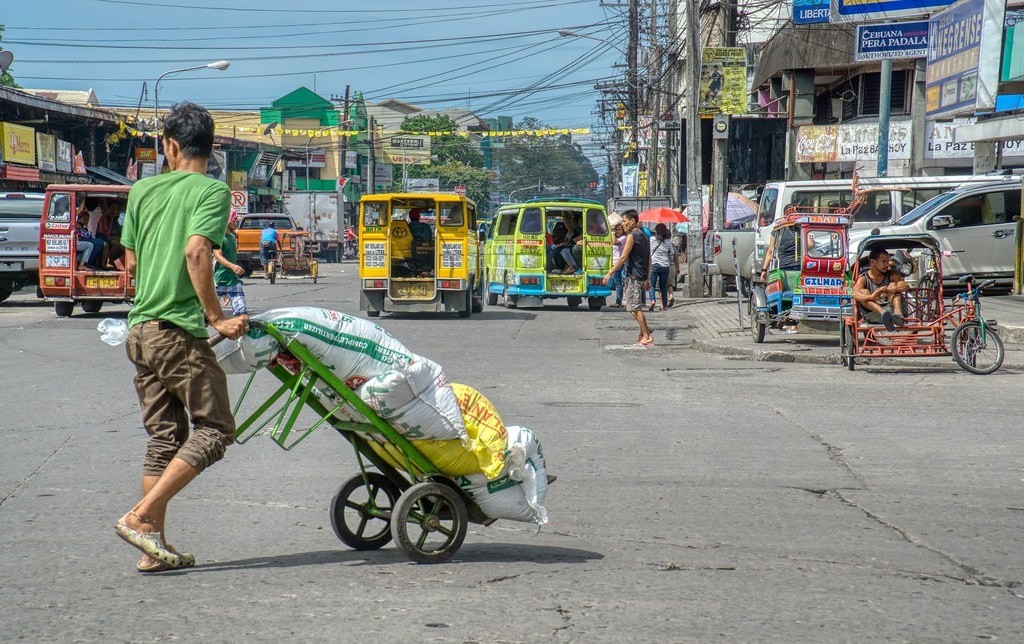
402,148,440,193
307,120,355,192
156,61,231,177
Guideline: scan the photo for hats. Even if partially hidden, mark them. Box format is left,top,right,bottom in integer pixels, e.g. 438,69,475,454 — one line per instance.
228,210,241,223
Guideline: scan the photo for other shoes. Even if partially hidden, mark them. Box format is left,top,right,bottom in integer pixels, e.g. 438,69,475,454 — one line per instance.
880,311,895,332
659,306,664,311
667,297,674,308
550,265,583,276
649,301,655,312
610,303,620,307
892,313,904,327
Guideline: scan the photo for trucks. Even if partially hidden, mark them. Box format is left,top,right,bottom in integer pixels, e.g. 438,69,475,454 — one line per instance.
607,194,675,246
280,192,345,265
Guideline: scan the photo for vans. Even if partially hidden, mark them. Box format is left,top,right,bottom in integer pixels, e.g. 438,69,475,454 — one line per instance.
754,174,1021,302
39,183,135,316
358,194,612,319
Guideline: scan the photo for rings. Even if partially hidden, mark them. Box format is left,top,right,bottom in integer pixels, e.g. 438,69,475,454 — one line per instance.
245,325,249,329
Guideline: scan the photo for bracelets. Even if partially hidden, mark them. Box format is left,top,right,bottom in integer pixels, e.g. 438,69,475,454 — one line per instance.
609,273,612,276
612,232,615,234
761,269,767,272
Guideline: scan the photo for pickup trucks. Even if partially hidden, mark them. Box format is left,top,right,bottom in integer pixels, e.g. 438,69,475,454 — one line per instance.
235,213,304,278
700,194,923,298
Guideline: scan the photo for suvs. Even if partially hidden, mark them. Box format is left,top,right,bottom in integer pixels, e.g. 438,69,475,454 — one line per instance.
0,192,56,302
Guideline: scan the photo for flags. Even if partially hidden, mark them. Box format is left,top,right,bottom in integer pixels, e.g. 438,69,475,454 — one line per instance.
106,120,590,145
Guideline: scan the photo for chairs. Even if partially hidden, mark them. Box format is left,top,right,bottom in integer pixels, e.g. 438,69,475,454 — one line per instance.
391,220,414,274
876,199,890,219
827,200,848,214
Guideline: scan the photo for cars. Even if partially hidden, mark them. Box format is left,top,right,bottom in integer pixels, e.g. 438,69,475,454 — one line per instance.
815,181,1022,288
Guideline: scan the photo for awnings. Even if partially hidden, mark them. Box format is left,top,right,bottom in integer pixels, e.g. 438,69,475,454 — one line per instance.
85,166,135,185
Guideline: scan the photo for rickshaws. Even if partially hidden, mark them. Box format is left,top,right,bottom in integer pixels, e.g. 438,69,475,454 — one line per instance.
268,231,318,285
748,166,865,343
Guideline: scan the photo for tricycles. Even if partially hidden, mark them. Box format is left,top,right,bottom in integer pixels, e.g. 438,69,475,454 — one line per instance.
838,232,1004,375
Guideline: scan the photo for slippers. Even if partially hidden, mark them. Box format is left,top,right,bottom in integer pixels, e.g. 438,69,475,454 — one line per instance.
782,327,798,334
634,340,655,347
636,327,652,342
136,551,195,572
113,524,186,569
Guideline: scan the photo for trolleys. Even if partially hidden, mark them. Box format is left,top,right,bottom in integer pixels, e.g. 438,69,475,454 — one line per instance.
205,319,557,564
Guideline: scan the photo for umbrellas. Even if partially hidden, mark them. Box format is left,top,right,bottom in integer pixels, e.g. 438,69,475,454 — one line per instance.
638,207,691,223
676,192,765,233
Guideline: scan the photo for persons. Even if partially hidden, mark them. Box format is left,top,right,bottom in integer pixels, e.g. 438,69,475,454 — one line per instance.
526,211,605,275
956,197,985,224
408,206,461,277
760,204,815,334
851,249,910,332
700,64,724,107
114,101,249,572
49,196,126,271
260,220,282,279
344,225,358,256
211,210,248,316
602,209,681,347
